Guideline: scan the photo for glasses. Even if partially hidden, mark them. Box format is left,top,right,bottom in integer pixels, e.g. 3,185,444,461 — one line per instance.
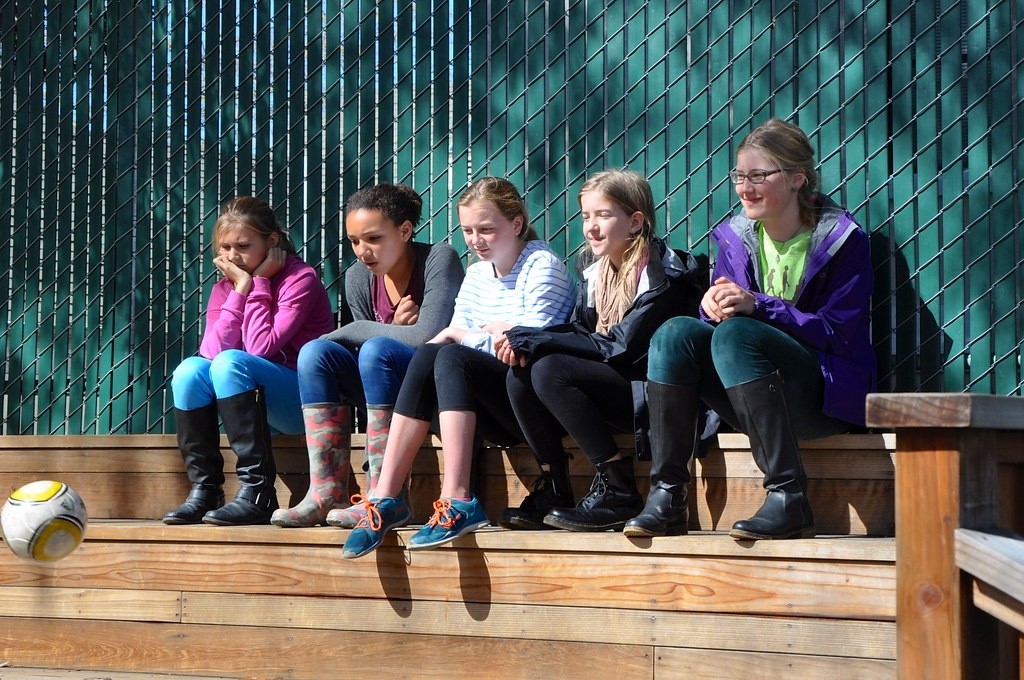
729,166,795,184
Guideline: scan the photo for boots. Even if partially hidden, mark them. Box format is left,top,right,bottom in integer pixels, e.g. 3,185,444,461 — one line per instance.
162,396,226,525
497,451,575,531
202,385,281,526
326,402,411,527
270,401,354,527
623,374,701,537
543,453,648,531
725,368,815,540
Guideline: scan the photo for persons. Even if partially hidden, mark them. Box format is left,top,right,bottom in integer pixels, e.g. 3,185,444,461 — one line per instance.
270,183,465,529
623,119,878,541
342,176,578,559
496,168,723,533
163,198,336,527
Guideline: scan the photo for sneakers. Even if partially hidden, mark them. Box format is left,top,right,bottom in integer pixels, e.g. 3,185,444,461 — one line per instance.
342,494,412,559
406,494,490,552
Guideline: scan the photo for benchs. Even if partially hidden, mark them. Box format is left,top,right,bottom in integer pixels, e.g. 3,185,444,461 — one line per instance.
0,434,896,535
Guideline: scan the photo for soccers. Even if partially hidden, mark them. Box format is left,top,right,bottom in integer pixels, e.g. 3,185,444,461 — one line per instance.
0,479,89,562
